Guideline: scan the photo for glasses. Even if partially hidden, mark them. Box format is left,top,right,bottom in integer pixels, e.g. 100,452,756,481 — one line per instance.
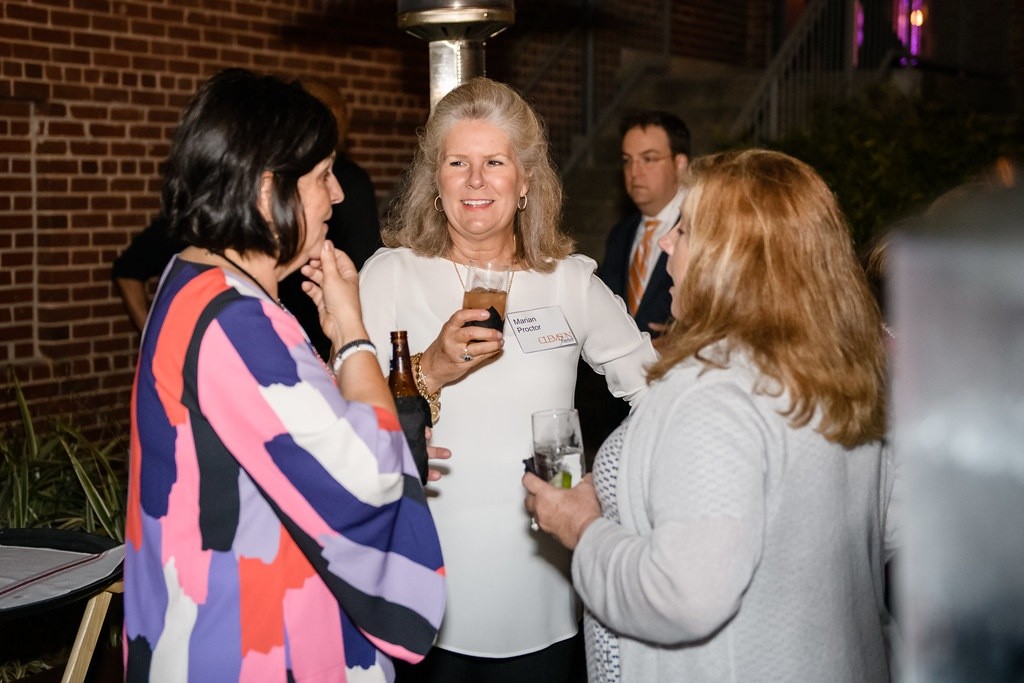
618,152,675,170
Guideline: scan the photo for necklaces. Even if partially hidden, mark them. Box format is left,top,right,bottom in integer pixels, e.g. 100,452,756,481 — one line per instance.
437,231,520,336
200,239,336,381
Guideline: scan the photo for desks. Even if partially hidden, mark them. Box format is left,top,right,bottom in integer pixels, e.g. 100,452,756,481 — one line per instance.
0,528,124,683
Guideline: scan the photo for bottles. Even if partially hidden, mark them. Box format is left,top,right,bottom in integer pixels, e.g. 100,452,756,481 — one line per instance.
388,331,429,485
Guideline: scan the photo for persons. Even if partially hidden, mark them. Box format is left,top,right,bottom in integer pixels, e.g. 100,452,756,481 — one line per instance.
114,67,383,400
519,141,968,683
349,74,669,683
112,65,450,683
579,107,703,485
868,109,1024,683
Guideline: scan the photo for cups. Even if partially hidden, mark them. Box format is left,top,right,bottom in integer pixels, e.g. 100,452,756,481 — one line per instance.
531,409,585,490
463,260,509,344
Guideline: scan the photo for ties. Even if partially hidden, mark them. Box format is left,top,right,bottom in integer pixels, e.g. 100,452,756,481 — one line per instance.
628,219,663,317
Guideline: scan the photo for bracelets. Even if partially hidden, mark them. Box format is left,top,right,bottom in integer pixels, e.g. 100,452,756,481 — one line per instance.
393,349,444,426
331,340,381,376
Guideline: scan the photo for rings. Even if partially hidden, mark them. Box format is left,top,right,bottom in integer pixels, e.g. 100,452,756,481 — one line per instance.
531,515,540,534
463,344,474,363
